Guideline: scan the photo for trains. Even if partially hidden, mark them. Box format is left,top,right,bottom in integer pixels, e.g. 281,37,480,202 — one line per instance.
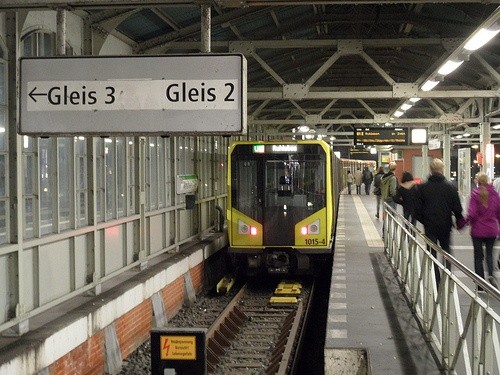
225,138,378,274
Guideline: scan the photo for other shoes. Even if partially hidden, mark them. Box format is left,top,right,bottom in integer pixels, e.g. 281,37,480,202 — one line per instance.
487,276,499,290
497,259,500,270
474,288,487,294
375,215,379,218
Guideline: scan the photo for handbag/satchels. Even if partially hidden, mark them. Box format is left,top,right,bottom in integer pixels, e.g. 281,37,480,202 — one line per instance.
374,187,380,195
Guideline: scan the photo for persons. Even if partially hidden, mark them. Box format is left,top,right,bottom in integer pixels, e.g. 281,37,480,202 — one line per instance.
354,167,363,195
346,171,354,194
373,166,385,218
465,171,500,291
408,158,466,290
393,172,417,242
380,161,397,216
362,167,373,195
